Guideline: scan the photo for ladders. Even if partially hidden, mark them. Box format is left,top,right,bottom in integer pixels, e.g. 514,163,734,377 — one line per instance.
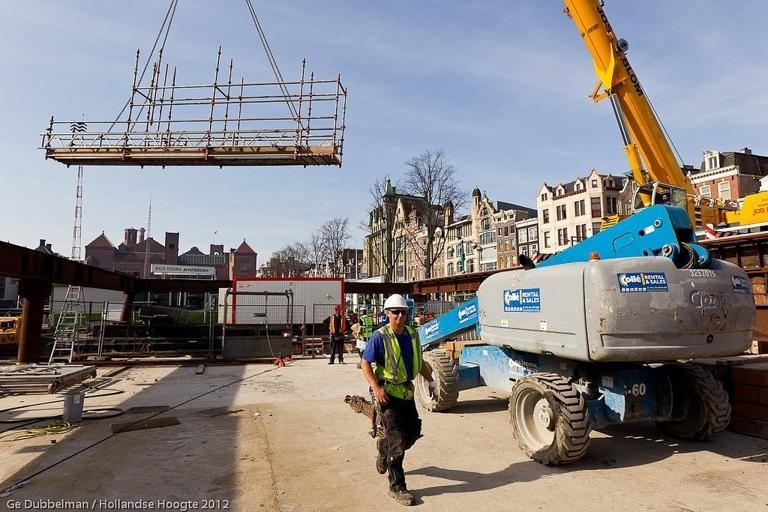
48,284,81,364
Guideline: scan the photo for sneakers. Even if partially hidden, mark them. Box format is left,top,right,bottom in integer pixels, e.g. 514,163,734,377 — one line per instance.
376,439,388,474
329,360,345,364
388,489,415,506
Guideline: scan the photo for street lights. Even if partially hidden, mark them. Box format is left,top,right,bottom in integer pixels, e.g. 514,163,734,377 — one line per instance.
447,235,481,274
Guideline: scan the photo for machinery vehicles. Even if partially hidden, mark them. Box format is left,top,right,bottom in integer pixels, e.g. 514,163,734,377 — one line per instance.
563,0,768,353
0,312,25,349
399,193,757,470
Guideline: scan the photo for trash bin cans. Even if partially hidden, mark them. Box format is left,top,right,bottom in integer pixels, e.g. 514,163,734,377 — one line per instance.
62,392,85,423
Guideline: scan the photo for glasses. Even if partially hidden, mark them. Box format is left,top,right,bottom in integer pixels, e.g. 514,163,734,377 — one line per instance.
388,309,406,315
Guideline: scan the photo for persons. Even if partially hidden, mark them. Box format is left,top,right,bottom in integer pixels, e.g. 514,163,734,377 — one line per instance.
322,304,351,365
359,292,439,506
349,315,371,369
359,306,375,342
660,192,670,206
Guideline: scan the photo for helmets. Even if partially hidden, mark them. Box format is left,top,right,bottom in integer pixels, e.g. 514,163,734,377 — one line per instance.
384,294,409,309
336,304,344,311
360,305,367,311
